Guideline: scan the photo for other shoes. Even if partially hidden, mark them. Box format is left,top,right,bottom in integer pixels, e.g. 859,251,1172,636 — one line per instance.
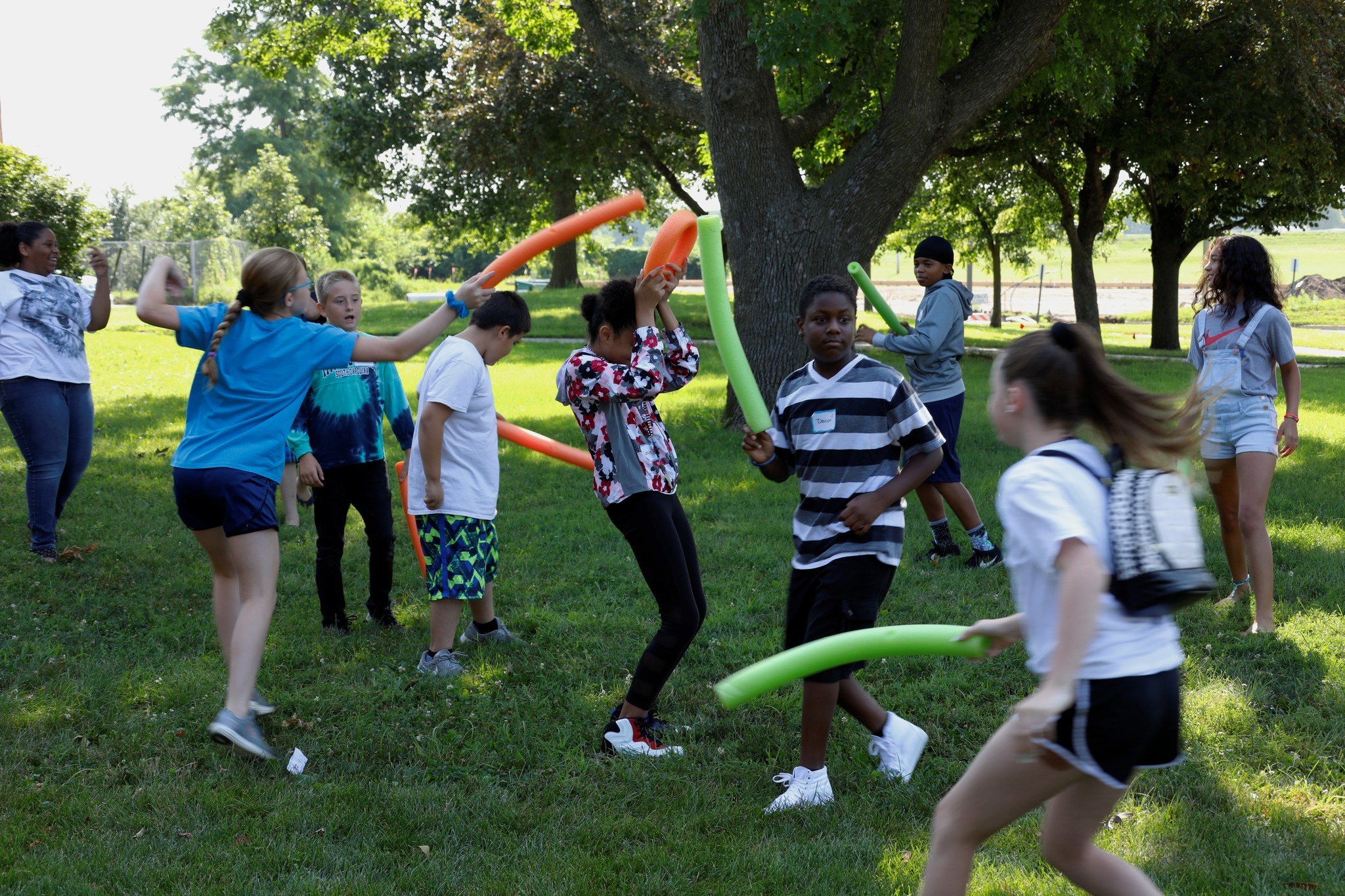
209,708,281,762
247,688,274,718
366,606,404,633
296,492,314,512
30,548,58,564
322,614,357,638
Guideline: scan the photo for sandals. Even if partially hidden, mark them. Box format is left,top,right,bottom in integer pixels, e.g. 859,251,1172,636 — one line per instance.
1213,574,1252,612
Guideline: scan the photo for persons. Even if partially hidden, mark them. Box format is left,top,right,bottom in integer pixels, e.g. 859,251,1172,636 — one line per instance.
921,320,1181,896
554,260,707,758
135,247,497,764
282,270,416,640
279,277,326,526
742,273,947,807
407,291,531,676
0,221,111,564
855,235,1005,571
1185,235,1300,637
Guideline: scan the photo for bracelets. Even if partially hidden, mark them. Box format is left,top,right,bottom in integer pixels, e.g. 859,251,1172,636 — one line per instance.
1284,413,1299,422
750,452,776,467
445,290,470,319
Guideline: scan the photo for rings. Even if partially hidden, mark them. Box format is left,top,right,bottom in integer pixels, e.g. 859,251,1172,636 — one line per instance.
1294,443,1298,446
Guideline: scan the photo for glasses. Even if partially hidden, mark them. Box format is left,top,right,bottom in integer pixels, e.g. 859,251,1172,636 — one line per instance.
280,275,313,306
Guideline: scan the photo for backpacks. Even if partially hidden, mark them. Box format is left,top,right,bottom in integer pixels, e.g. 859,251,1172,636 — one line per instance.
1033,442,1215,618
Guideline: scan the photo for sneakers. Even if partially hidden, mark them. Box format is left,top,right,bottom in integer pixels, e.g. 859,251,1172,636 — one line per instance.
601,702,692,759
914,540,961,563
459,616,530,648
961,543,1005,571
867,710,929,785
762,765,834,816
416,649,468,678
